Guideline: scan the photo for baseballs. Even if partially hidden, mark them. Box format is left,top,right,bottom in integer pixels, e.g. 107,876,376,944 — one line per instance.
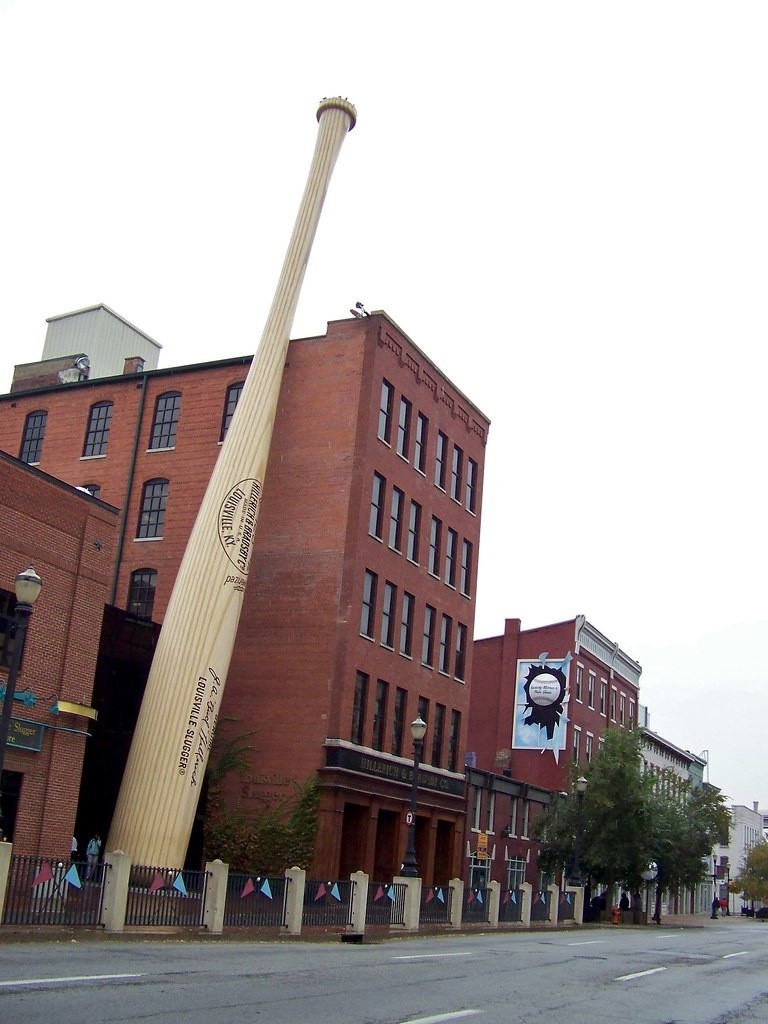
529,673,561,706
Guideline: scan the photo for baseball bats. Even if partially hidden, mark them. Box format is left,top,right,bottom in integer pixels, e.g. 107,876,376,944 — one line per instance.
102,96,358,889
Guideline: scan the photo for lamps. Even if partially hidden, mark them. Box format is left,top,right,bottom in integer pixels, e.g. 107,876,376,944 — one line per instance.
14,687,34,707
0,679,6,702
30,695,59,717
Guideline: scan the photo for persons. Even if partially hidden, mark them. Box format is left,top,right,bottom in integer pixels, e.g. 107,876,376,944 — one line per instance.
720,898,728,917
713,897,720,915
70,837,78,862
599,893,606,910
83,833,102,880
620,893,629,910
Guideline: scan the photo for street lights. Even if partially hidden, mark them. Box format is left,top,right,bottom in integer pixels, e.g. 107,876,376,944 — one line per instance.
398,712,427,877
0,562,44,773
725,863,732,915
